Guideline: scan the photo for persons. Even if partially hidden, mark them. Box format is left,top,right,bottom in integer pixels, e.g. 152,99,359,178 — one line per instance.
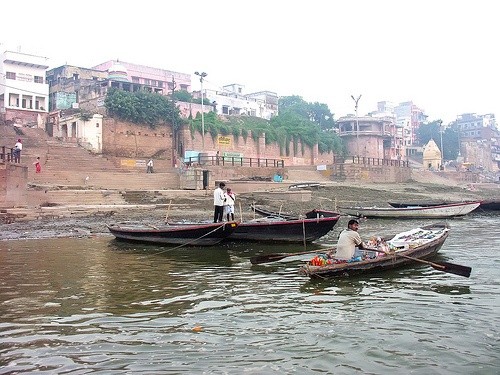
335,219,364,262
214,182,225,223
35,157,41,173
223,188,235,223
13,138,22,160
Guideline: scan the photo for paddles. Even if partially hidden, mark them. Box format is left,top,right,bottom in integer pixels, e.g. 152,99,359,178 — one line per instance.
250,247,337,264
358,244,473,279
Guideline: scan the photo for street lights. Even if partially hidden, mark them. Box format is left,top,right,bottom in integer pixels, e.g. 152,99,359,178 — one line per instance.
350,94,363,157
438,118,444,165
194,70,208,151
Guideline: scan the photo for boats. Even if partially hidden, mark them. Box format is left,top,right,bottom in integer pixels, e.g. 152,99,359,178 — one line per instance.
248,203,306,220
387,199,500,213
297,221,450,279
336,201,483,219
104,210,340,247
